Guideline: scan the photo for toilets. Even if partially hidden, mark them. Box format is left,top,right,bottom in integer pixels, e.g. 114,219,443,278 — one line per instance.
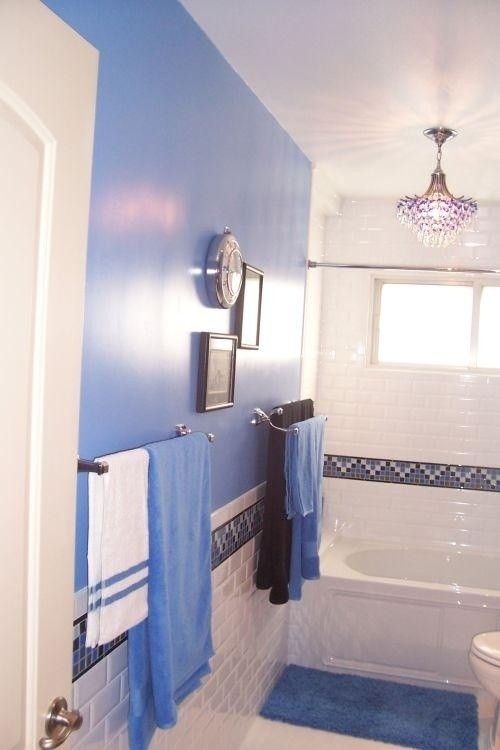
468,630,500,750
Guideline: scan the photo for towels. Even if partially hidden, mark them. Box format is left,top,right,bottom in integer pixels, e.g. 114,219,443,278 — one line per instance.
122,433,217,749
85,447,149,651
254,396,316,605
282,417,325,601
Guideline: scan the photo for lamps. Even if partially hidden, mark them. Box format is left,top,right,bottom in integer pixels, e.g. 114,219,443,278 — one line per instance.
393,123,480,251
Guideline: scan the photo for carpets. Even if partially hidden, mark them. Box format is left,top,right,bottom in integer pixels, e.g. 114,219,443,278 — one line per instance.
255,663,484,750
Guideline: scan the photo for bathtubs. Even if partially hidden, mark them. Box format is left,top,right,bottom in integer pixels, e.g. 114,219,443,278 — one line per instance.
284,531,500,695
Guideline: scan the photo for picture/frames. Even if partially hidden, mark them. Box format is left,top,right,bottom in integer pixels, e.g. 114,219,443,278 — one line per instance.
196,329,238,415
233,262,267,352
